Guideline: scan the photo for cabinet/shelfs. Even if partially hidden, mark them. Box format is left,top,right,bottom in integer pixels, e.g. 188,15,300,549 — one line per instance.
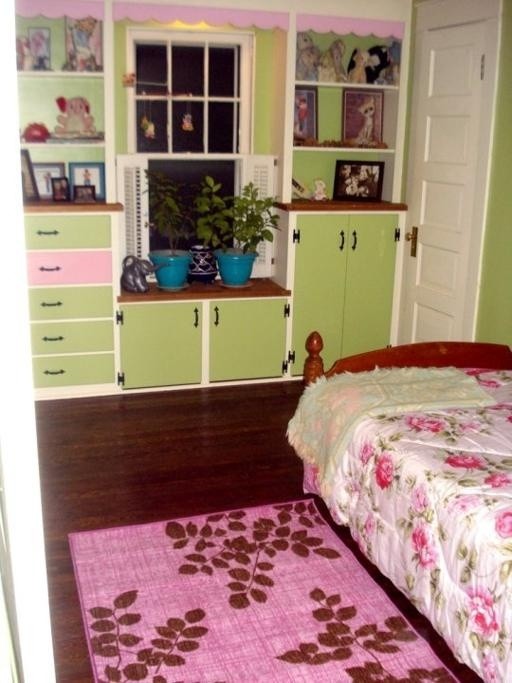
113,278,290,395
271,203,408,383
18,1,117,207
22,202,125,401
271,0,413,206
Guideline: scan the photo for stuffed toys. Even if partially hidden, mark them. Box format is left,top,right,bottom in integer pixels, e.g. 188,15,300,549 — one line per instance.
55,97,94,132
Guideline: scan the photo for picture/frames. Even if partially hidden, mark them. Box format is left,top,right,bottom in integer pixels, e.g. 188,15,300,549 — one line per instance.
21,148,106,205
294,85,319,142
341,85,385,146
331,158,385,205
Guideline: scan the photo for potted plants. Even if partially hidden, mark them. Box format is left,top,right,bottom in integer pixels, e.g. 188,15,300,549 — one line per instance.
135,169,281,291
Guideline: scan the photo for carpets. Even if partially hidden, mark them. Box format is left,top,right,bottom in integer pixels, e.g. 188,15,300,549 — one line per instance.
65,496,459,683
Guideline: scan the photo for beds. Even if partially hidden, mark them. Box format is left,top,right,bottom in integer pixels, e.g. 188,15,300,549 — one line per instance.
301,327,512,683
